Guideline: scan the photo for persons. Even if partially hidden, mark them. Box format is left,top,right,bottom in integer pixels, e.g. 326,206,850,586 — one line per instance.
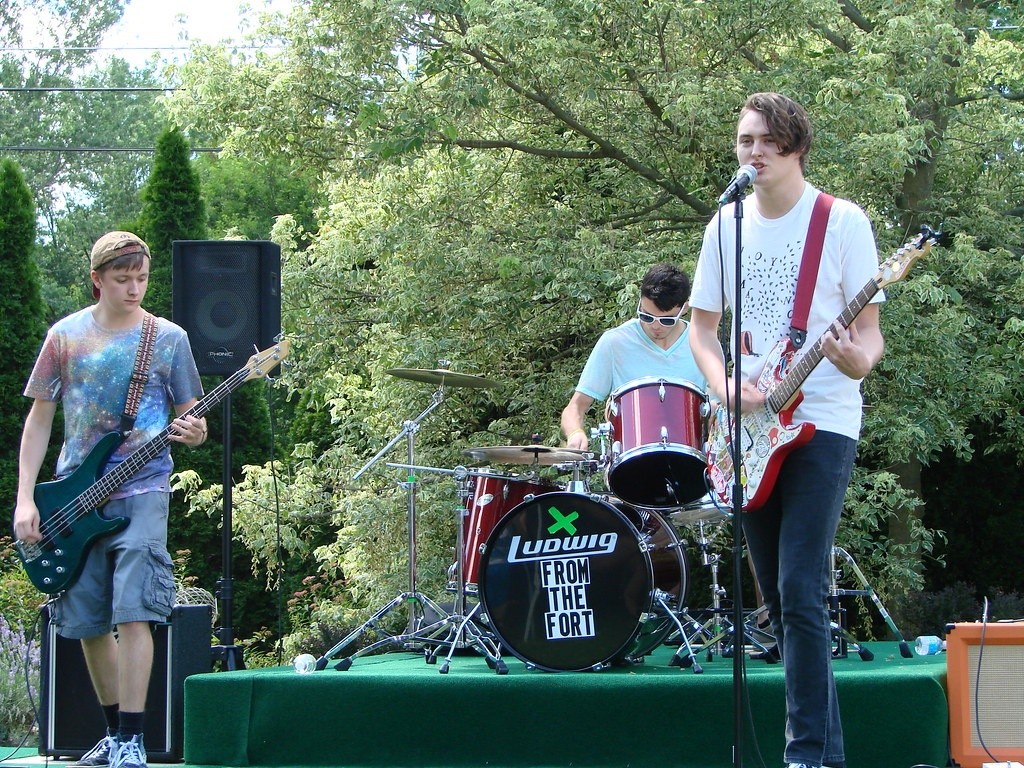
12,230,208,768
562,264,777,647
689,93,885,768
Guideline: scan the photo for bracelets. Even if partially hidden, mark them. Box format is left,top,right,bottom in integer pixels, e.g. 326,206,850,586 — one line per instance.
566,429,584,439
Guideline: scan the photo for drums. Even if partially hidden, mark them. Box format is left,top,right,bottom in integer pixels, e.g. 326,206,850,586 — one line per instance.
447,468,567,588
476,492,691,672
668,441,734,523
604,375,711,510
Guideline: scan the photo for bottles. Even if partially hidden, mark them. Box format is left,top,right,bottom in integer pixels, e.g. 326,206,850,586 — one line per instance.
294,654,317,674
915,635,946,655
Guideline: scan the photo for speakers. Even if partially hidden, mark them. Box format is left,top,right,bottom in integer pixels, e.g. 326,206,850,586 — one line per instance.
38,604,213,763
171,239,282,378
946,621,1024,768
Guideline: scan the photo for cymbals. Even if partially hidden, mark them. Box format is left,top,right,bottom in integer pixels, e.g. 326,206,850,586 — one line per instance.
862,405,875,409
384,368,498,389
459,444,601,465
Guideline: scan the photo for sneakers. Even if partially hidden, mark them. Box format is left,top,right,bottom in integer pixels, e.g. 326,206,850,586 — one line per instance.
110,733,148,768
76,727,117,765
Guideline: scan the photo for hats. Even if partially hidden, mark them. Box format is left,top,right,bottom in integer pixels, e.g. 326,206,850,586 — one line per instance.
91,231,150,299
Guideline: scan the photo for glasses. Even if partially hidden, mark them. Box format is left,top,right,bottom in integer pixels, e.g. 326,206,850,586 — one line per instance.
637,299,686,327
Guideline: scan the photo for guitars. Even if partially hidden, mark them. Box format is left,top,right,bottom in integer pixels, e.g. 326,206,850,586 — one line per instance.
14,336,290,595
706,230,931,510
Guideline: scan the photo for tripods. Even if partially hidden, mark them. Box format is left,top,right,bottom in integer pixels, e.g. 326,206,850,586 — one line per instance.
312,382,539,678
666,519,915,672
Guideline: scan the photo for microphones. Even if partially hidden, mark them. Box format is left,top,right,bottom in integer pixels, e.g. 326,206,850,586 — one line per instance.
719,164,758,205
